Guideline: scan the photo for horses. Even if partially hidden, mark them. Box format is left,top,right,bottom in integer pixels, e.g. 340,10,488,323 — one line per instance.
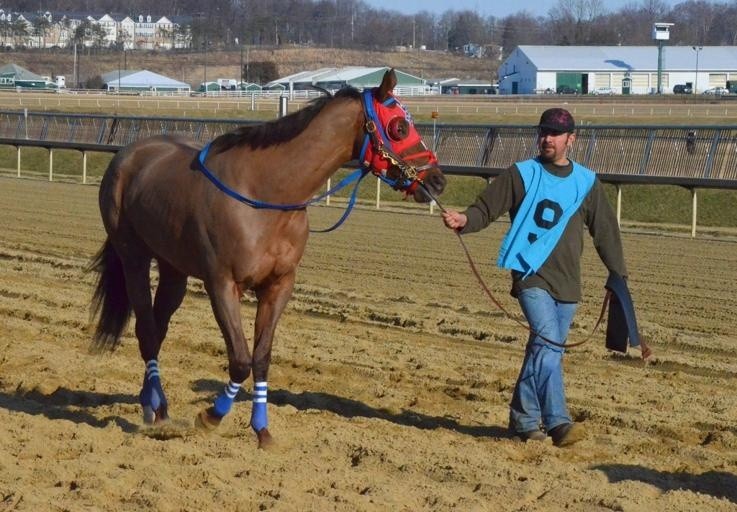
80,64,449,454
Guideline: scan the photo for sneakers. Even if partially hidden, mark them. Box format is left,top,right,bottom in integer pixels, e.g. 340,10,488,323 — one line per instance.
551,421,588,446
507,423,546,443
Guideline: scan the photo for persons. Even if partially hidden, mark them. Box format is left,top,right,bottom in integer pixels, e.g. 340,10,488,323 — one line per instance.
440,108,628,447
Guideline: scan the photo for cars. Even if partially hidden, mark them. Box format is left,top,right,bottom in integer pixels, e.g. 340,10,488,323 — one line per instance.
674,85,692,94
557,85,578,95
705,88,729,96
592,87,617,96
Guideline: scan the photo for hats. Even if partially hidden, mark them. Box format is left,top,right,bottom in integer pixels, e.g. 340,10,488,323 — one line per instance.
539,108,574,133
